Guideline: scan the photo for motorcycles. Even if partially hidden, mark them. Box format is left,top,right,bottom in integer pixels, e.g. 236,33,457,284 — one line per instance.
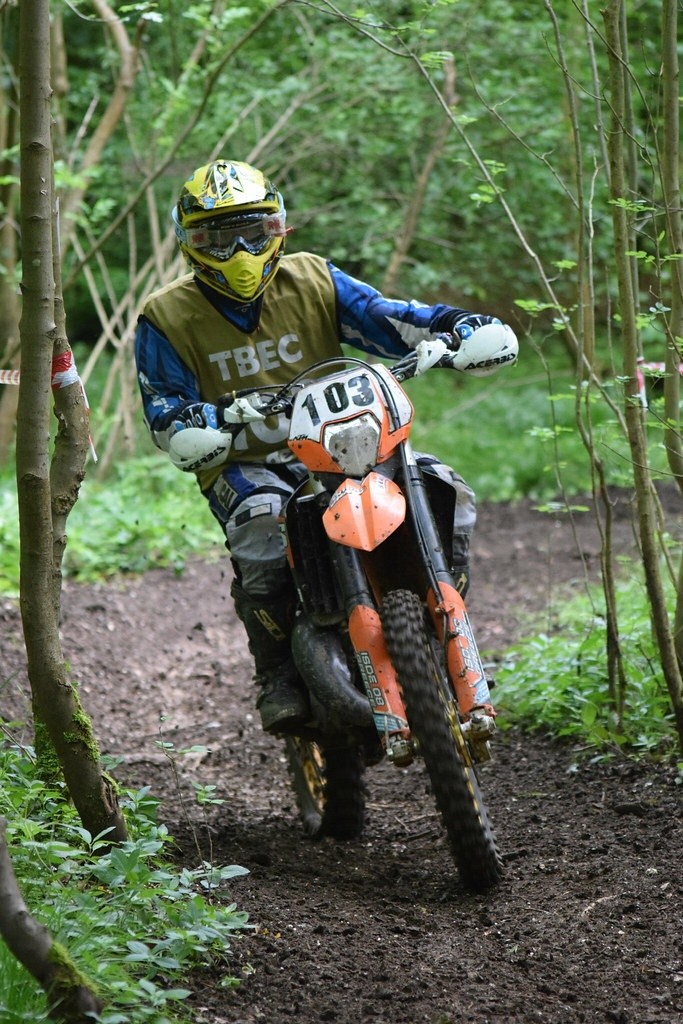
168,324,510,897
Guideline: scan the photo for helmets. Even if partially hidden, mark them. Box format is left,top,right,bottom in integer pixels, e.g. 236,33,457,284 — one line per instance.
175,160,286,304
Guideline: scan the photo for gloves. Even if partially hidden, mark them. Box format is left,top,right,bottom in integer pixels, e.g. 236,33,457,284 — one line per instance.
171,402,218,433
452,313,503,346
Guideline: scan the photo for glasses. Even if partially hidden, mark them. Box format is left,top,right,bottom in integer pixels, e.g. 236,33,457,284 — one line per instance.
172,192,283,260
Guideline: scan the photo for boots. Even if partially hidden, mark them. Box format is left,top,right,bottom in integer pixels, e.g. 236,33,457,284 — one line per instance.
448,563,471,600
231,577,312,731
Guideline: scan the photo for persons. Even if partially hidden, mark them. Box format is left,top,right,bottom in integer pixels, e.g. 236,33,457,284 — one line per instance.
132,157,505,731
